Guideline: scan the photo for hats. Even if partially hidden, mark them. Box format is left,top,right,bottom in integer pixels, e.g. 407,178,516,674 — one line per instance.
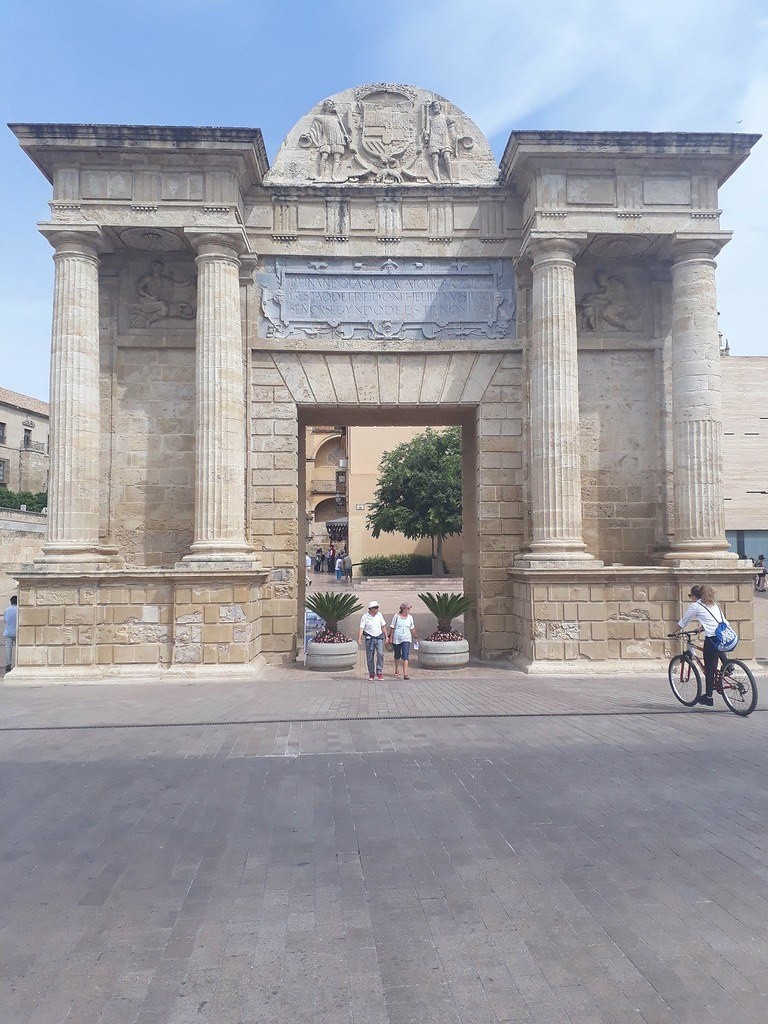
758,555,765,560
368,601,381,609
400,602,412,609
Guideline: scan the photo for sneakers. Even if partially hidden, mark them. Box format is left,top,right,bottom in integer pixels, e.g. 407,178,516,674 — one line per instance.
376,674,384,680
369,674,374,680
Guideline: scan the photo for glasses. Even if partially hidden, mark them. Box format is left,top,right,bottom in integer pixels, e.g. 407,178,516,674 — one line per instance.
372,606,378,609
688,594,692,598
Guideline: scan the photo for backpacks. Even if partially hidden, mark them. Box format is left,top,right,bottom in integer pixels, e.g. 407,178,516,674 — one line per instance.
695,602,738,652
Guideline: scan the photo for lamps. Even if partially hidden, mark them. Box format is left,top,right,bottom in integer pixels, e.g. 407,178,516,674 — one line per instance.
306,509,315,522
333,493,348,517
340,459,348,468
339,475,347,488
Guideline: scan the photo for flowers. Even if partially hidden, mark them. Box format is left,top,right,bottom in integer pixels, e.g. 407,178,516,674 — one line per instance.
425,629,465,642
313,630,353,643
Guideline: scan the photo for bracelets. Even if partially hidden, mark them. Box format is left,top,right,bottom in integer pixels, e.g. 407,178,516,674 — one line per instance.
385,638,389,640
414,637,417,639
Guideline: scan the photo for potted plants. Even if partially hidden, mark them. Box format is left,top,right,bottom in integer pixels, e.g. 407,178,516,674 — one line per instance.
417,590,475,671
303,591,365,673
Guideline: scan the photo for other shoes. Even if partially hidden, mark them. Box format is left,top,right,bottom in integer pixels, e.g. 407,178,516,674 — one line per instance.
721,664,735,673
759,589,766,592
404,675,410,679
337,580,341,583
309,581,312,586
395,671,400,675
697,694,714,706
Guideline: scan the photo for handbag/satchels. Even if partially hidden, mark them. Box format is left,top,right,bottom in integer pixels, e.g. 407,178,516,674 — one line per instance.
385,635,393,652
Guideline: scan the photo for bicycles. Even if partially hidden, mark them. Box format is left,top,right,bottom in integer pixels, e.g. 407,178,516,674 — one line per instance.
667,629,759,717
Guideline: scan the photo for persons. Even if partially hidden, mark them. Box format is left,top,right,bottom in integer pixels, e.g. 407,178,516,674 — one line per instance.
3,596,23,673
358,601,388,681
423,100,458,183
581,270,641,331
390,602,418,679
742,555,767,592
305,545,352,586
671,585,735,706
128,261,193,329
302,99,351,181
